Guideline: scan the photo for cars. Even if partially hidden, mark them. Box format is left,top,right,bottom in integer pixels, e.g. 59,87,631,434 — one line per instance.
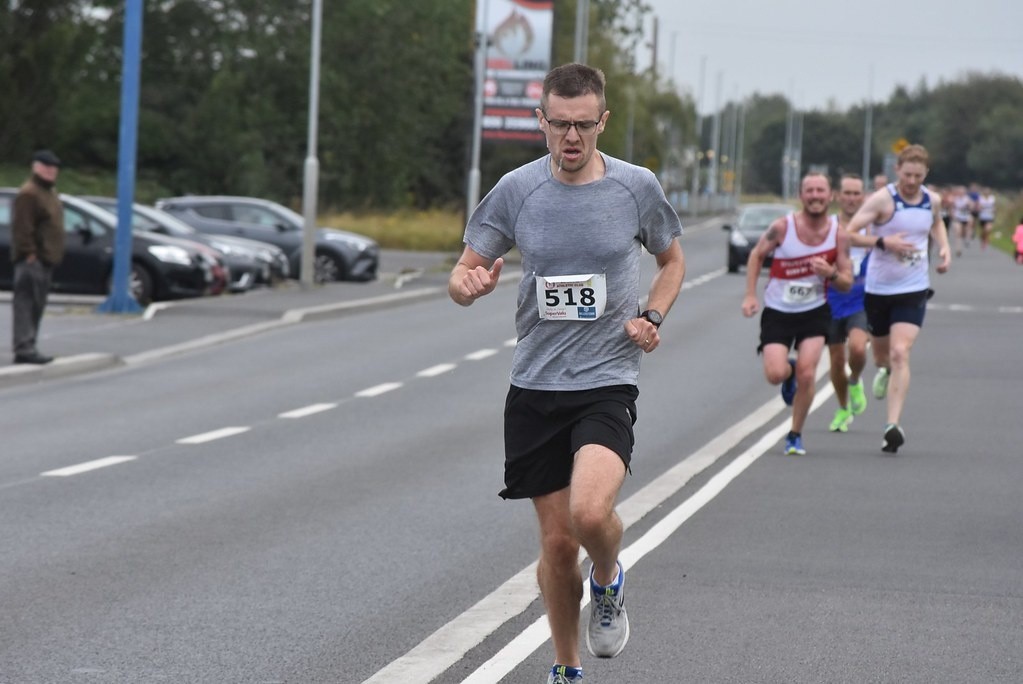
154,197,381,288
70,194,290,296
722,203,798,275
1,188,213,307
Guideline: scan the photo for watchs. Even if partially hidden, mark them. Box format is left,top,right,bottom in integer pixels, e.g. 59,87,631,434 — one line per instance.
641,309,662,329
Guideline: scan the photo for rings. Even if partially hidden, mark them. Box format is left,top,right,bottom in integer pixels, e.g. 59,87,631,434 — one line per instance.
645,339,651,345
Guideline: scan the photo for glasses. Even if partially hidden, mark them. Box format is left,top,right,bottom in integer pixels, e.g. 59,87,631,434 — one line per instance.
542,114,601,136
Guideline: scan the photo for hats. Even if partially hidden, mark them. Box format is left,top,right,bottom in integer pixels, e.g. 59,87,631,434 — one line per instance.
31,150,60,181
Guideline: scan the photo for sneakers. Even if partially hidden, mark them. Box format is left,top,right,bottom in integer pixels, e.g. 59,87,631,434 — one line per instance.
546,661,583,684
586,558,630,658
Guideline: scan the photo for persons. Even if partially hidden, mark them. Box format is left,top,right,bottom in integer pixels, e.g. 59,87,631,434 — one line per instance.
448,63,685,684
928,183,997,259
846,145,949,452
1011,217,1023,264
9,152,66,366
824,172,889,431
741,165,853,456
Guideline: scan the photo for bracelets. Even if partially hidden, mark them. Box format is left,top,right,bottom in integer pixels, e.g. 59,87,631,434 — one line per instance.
829,270,838,282
876,236,885,252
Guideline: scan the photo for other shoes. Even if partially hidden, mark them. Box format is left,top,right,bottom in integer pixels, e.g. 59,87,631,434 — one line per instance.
781,354,798,406
783,437,806,456
14,350,53,364
847,375,867,416
829,404,854,432
881,423,906,453
871,367,889,399
